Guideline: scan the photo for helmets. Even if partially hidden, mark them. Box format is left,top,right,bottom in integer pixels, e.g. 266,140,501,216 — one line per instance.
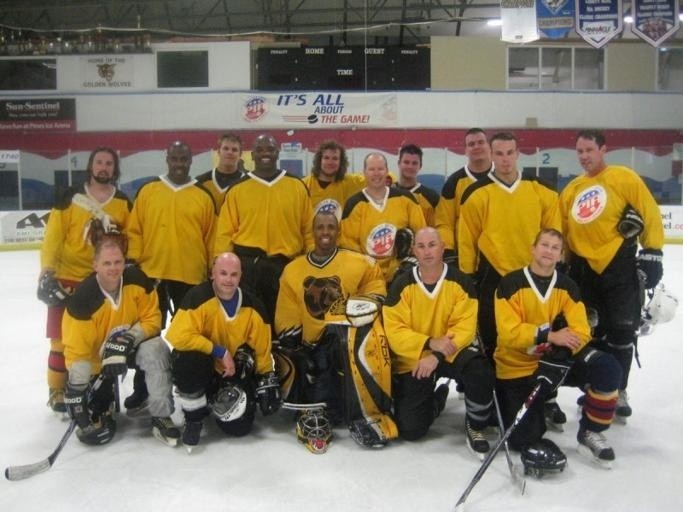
209,385,254,437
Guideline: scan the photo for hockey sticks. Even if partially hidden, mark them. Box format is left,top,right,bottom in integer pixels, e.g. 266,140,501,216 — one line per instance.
5,374,106,481
454,381,542,512
476,326,526,494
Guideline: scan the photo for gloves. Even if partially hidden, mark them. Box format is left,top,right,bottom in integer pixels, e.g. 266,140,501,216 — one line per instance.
257,372,280,416
616,205,644,240
101,331,133,377
636,247,663,290
234,343,255,381
62,390,88,417
395,228,414,259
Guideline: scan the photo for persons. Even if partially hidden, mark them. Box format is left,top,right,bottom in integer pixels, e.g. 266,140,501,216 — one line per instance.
559,131,663,419
298,139,396,250
38,148,133,412
433,128,495,270
162,252,280,453
192,134,248,216
273,210,398,454
382,225,494,460
393,144,438,225
340,153,427,288
454,130,567,433
491,228,622,468
60,217,180,447
123,139,218,415
213,134,314,317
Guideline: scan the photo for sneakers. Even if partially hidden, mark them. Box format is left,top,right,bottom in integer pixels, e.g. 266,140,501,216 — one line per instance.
48,387,67,413
123,388,148,409
577,428,614,460
544,402,565,424
151,416,180,438
465,415,489,453
613,390,632,417
434,383,448,412
181,422,202,445
